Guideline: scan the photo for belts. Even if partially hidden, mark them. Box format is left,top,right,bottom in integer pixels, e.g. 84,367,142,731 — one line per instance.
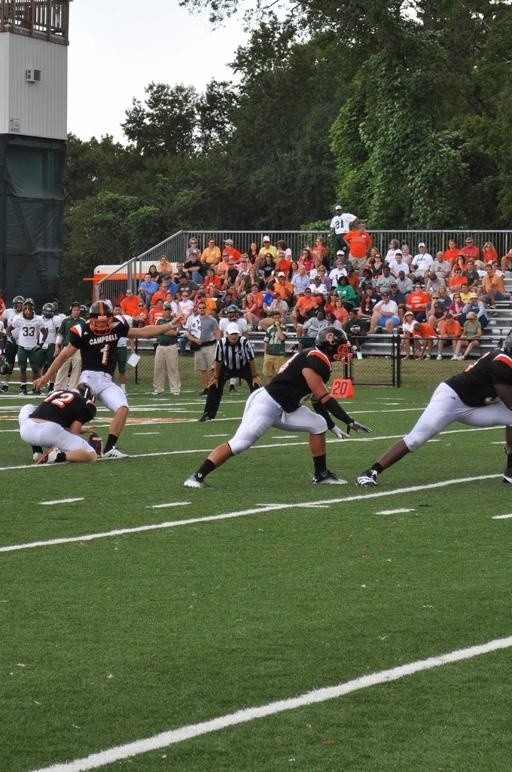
159,342,175,346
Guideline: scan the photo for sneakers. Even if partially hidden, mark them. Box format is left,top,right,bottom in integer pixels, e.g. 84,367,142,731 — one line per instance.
401,351,465,362
311,469,349,485
500,467,512,483
353,470,379,489
0,384,46,396
32,446,62,465
198,412,213,422
152,387,236,396
102,446,129,460
183,473,220,491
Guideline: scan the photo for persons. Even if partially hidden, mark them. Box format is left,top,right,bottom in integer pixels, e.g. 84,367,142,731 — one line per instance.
330,206,359,265
113,306,130,393
264,311,288,383
120,236,512,359
342,222,372,269
99,293,112,306
19,382,99,465
187,302,222,395
356,334,512,488
198,324,263,422
0,296,88,395
152,303,182,395
33,300,183,457
184,326,372,488
219,305,248,392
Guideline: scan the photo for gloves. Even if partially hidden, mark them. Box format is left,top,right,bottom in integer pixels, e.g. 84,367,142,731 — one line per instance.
346,420,373,435
330,424,350,440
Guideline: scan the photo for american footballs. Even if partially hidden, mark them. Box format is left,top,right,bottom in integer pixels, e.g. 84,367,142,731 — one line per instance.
89,433,103,458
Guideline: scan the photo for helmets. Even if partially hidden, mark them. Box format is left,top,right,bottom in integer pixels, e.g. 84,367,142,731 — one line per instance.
42,303,55,317
227,304,241,321
89,301,114,336
13,296,35,313
79,304,88,317
313,326,353,365
502,330,512,356
75,382,97,403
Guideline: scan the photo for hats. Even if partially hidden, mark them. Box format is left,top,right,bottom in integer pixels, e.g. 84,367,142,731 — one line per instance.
418,242,425,248
335,205,342,211
223,239,234,246
226,322,240,335
469,293,478,300
162,302,171,310
336,250,344,256
263,236,270,244
278,271,285,278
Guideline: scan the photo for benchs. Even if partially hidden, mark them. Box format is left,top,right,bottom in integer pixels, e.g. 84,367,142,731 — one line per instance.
126,270,512,357
